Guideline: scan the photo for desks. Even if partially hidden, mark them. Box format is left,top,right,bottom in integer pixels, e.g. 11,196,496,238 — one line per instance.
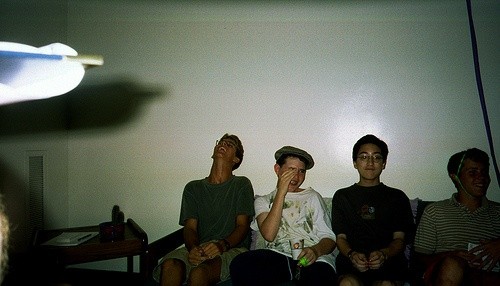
30,219,148,271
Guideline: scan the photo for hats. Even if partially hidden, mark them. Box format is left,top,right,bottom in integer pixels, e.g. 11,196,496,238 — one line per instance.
275,146,315,170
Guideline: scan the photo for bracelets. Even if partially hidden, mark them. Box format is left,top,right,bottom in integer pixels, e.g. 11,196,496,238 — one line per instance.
378,249,386,261
348,249,354,258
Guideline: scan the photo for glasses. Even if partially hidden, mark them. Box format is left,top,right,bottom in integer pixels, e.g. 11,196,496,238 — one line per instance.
215,139,237,148
357,155,383,162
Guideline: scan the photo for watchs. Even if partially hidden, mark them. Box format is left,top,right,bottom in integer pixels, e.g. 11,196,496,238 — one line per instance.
223,239,230,252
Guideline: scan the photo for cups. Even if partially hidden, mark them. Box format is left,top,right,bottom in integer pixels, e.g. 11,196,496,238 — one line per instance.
288,238,304,260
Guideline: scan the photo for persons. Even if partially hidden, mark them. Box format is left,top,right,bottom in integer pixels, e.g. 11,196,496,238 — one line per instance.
152,134,255,286
409,148,500,286
228,146,338,286
331,135,414,286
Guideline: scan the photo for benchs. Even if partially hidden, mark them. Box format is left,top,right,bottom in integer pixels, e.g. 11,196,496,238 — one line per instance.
142,201,436,286
